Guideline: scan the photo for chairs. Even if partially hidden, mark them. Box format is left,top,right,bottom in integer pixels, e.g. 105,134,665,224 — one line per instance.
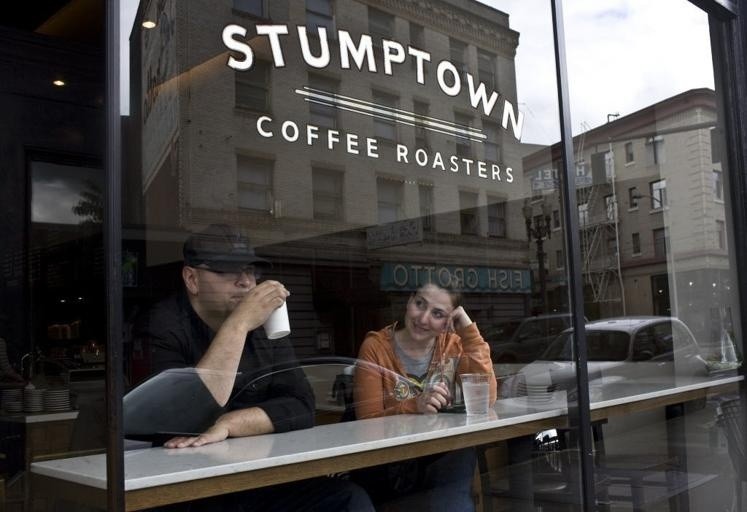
330,375,355,419
470,375,746,512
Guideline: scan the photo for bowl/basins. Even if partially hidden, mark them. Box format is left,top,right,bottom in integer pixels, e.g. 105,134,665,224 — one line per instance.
80,352,105,363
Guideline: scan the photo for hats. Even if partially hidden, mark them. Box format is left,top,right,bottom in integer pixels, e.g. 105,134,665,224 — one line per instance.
183,224,273,280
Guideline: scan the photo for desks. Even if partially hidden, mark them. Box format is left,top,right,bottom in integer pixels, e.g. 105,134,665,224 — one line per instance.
29,371,747,512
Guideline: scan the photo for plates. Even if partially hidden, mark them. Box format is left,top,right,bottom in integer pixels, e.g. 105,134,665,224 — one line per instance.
0,385,71,413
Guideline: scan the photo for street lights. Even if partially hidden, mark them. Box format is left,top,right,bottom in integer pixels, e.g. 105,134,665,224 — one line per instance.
519,190,554,317
631,191,679,321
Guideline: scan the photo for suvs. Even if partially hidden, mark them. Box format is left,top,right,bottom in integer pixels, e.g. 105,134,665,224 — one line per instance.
488,314,591,364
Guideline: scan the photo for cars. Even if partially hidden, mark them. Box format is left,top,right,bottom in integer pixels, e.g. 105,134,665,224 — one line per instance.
123,353,453,498
497,314,712,450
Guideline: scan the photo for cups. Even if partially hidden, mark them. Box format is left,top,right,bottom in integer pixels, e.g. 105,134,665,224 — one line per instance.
262,302,291,341
457,371,492,416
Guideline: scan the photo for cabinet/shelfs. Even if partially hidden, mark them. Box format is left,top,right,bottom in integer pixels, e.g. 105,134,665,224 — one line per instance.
0,409,80,467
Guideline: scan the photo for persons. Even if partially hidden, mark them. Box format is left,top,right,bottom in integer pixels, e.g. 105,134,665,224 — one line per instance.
134,221,378,512
705,325,740,422
342,276,499,509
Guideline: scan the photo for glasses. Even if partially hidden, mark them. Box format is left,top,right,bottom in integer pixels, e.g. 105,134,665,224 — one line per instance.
195,265,265,281
